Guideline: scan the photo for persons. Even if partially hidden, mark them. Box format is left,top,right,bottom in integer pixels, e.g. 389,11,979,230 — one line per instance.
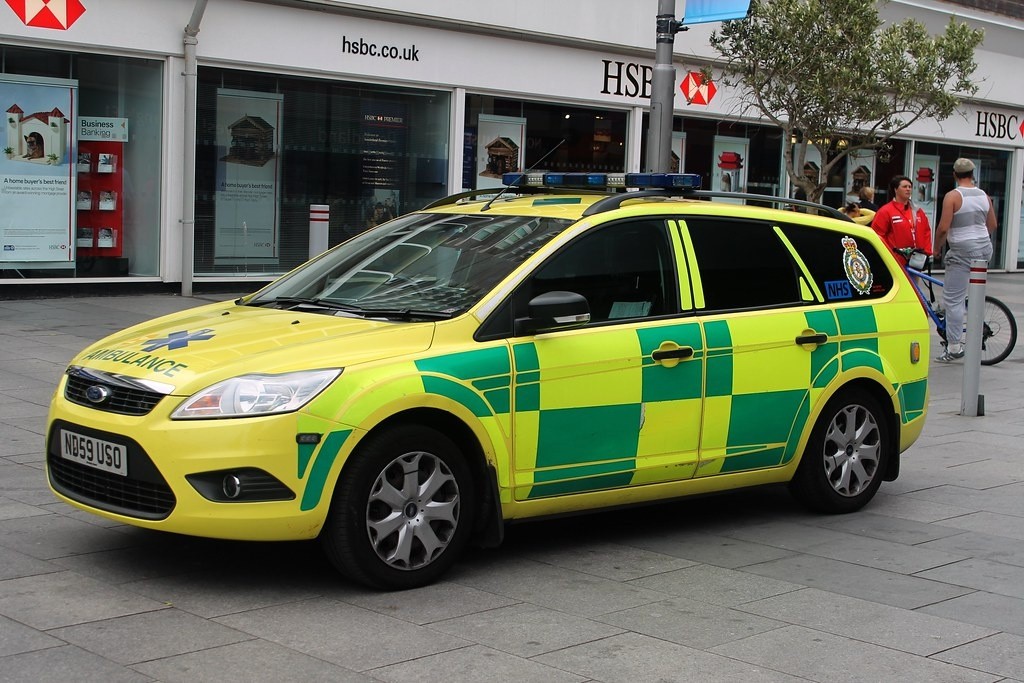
795,189,808,201
933,158,996,361
872,175,933,284
838,203,876,225
858,186,878,226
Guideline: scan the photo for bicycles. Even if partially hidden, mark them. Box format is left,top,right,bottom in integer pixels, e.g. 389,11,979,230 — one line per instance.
892,245,1019,367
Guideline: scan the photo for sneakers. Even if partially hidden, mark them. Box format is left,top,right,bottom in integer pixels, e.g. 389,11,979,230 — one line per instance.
934,349,964,362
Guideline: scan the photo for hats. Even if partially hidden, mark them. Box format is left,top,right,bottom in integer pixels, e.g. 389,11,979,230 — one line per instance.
953,158,975,173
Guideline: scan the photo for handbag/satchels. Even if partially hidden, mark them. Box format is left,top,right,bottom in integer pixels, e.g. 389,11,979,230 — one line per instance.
908,249,935,303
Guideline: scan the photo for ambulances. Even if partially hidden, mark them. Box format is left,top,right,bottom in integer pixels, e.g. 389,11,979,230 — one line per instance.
40,168,937,595
